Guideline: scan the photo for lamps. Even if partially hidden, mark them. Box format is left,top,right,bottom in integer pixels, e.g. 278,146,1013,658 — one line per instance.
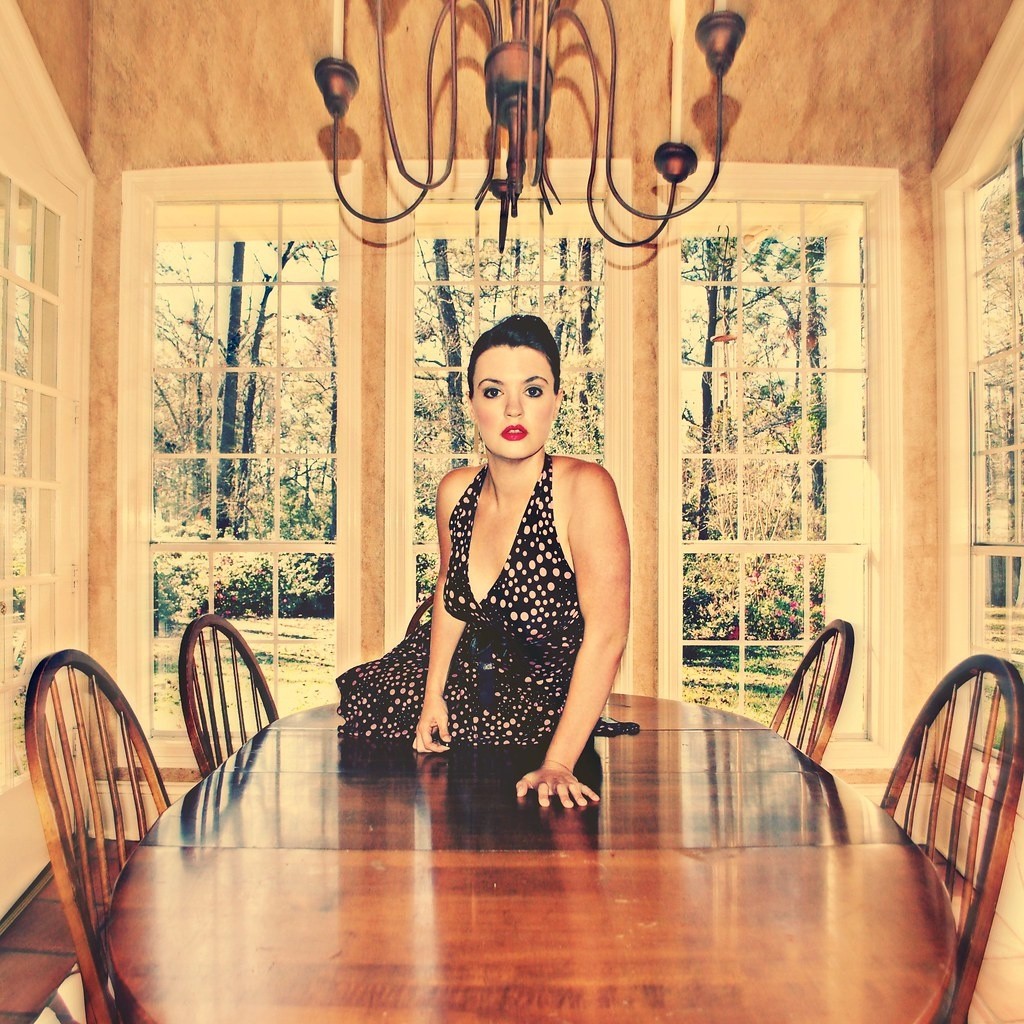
315,0,748,253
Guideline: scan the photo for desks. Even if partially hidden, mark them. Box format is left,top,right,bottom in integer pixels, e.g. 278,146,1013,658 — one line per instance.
110,693,958,1024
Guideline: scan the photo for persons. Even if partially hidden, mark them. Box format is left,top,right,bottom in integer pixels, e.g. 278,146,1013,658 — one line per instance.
336,314,630,809
337,734,603,888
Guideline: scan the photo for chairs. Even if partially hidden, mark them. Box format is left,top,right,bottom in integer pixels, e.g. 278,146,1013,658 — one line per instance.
23,649,170,1024
178,614,280,777
879,655,1024,1024
771,620,856,760
